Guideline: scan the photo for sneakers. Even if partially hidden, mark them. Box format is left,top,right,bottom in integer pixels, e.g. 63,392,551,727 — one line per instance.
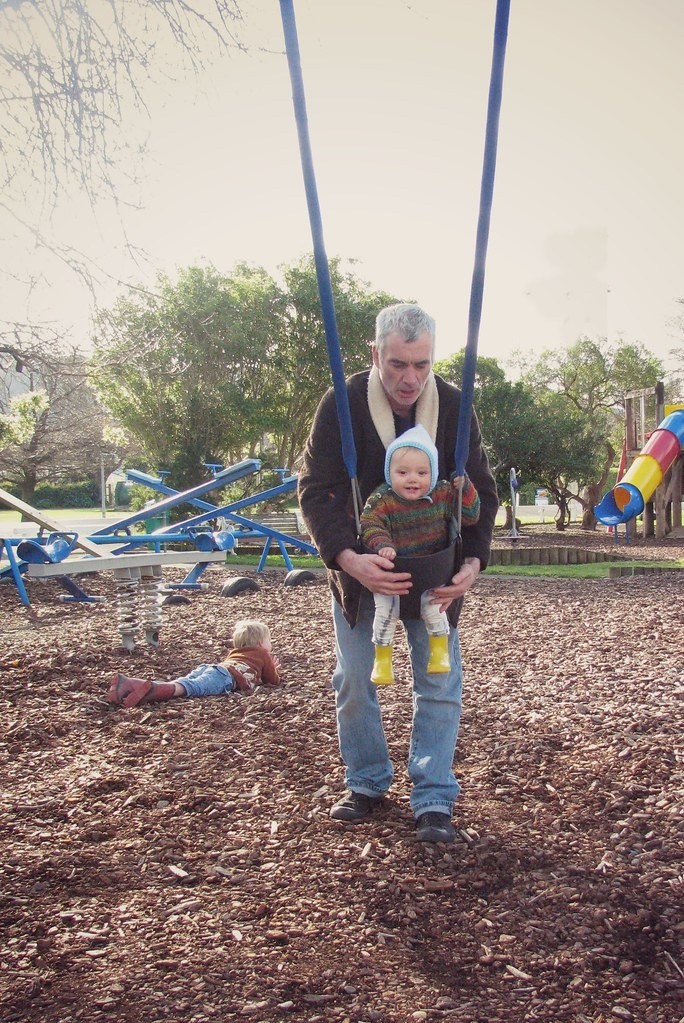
330,790,379,818
417,810,456,842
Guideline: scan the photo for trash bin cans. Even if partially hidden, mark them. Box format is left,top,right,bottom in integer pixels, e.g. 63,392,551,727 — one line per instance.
144,502,171,551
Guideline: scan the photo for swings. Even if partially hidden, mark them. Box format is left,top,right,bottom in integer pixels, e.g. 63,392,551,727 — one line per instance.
280,1,512,622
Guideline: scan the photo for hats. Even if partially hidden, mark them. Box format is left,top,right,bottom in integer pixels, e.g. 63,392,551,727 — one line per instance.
384,423,439,504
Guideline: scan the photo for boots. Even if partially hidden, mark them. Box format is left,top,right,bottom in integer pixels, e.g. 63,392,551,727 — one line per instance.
370,644,395,685
426,633,451,675
107,673,153,705
124,680,175,708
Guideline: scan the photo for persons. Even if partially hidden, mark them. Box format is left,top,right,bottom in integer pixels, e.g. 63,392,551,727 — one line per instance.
108,620,281,707
360,422,480,684
298,303,500,843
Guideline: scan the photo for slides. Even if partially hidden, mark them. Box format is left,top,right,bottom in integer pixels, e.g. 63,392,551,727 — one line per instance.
591,411,683,526
